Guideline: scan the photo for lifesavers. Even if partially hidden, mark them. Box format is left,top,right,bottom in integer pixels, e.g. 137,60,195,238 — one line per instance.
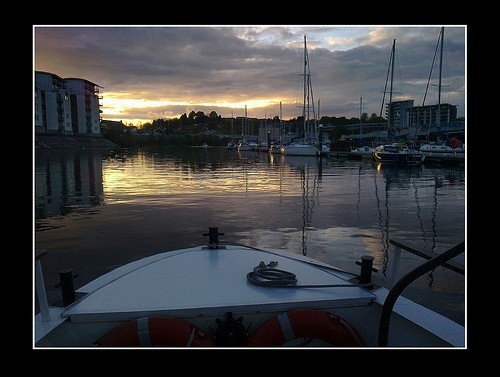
246,309,366,348
95,315,213,348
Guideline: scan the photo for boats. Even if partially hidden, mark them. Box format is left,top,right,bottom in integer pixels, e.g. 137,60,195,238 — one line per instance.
238,142,258,151
372,145,422,165
281,143,321,155
422,144,459,157
33,224,465,346
